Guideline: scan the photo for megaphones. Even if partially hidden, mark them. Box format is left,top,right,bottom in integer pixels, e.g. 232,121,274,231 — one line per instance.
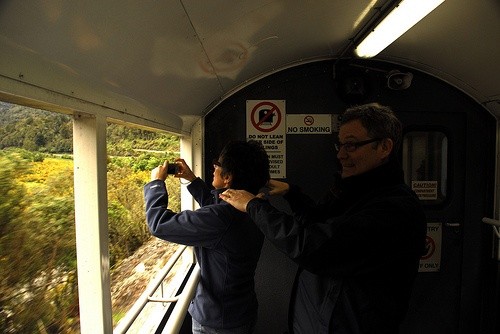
386,70,411,90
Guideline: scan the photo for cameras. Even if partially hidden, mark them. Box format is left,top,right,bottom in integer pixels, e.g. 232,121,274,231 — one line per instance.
167,163,179,174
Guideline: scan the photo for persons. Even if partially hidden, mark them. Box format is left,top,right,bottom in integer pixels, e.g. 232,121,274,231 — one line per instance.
218,103,425,334
144,137,270,334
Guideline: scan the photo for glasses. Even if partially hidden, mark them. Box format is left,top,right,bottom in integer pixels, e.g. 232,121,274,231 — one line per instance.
335,138,378,152
211,158,221,167
